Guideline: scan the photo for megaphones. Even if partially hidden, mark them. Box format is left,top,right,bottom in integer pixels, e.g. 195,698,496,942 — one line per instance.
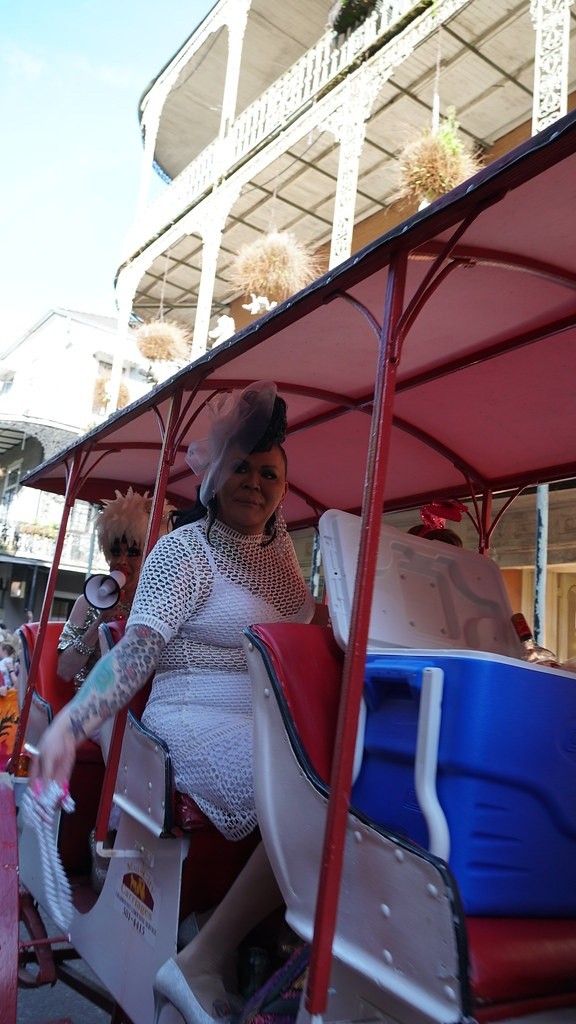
82,569,130,610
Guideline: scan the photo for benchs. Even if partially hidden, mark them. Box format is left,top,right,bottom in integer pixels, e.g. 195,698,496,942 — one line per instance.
16,621,103,896
92,619,257,955
242,622,576,1024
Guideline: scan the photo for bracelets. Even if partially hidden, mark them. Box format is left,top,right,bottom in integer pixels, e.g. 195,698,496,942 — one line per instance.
74,635,95,655
9,670,14,673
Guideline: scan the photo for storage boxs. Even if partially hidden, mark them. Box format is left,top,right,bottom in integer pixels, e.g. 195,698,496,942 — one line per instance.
317,506,575,921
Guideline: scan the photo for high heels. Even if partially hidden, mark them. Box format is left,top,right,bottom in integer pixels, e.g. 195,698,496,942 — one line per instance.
153,957,242,1024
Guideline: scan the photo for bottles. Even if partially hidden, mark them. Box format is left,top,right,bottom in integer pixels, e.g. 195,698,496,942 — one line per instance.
511,613,559,668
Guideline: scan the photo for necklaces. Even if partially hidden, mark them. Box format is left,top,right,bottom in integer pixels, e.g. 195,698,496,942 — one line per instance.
23,781,77,929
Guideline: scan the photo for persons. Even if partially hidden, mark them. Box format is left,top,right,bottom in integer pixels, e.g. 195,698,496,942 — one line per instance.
406,525,463,548
0,610,33,697
26,379,317,1024
56,490,177,893
